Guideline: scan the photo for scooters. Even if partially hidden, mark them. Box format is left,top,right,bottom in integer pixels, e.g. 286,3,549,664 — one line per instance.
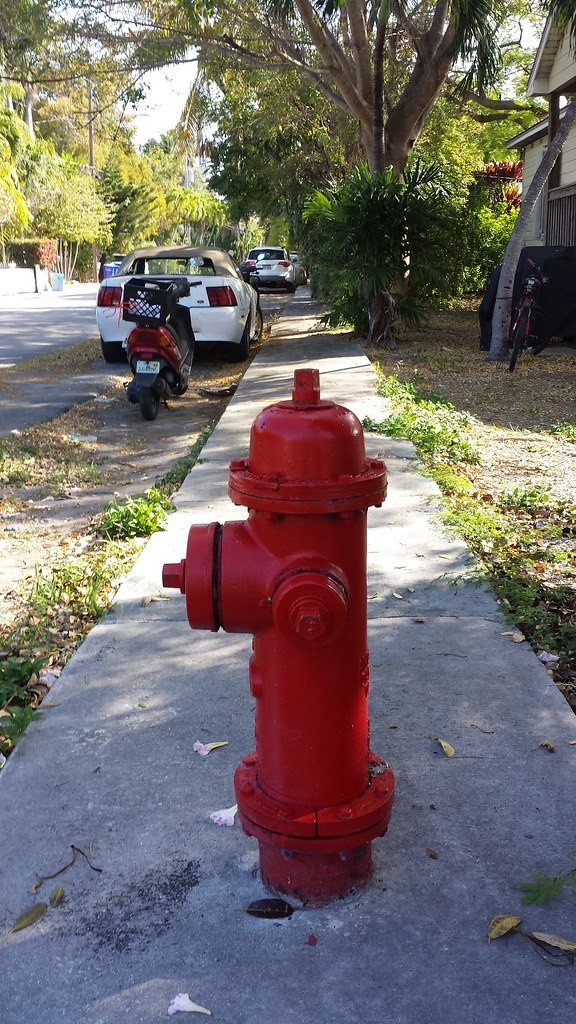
118,256,205,420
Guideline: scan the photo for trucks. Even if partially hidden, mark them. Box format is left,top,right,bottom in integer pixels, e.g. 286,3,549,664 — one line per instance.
103,254,126,282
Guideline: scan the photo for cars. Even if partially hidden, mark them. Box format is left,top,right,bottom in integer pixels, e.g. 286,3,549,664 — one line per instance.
96,245,265,363
287,250,309,285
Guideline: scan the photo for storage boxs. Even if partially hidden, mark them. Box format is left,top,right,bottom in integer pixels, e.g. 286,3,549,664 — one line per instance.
121,277,175,327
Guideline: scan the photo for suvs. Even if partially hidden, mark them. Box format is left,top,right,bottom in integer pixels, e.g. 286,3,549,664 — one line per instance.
241,244,297,295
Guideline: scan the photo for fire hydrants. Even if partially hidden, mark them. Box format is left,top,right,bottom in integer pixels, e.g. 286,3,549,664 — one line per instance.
159,368,395,914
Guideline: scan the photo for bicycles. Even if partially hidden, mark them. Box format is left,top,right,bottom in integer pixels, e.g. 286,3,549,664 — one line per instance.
507,256,551,372
